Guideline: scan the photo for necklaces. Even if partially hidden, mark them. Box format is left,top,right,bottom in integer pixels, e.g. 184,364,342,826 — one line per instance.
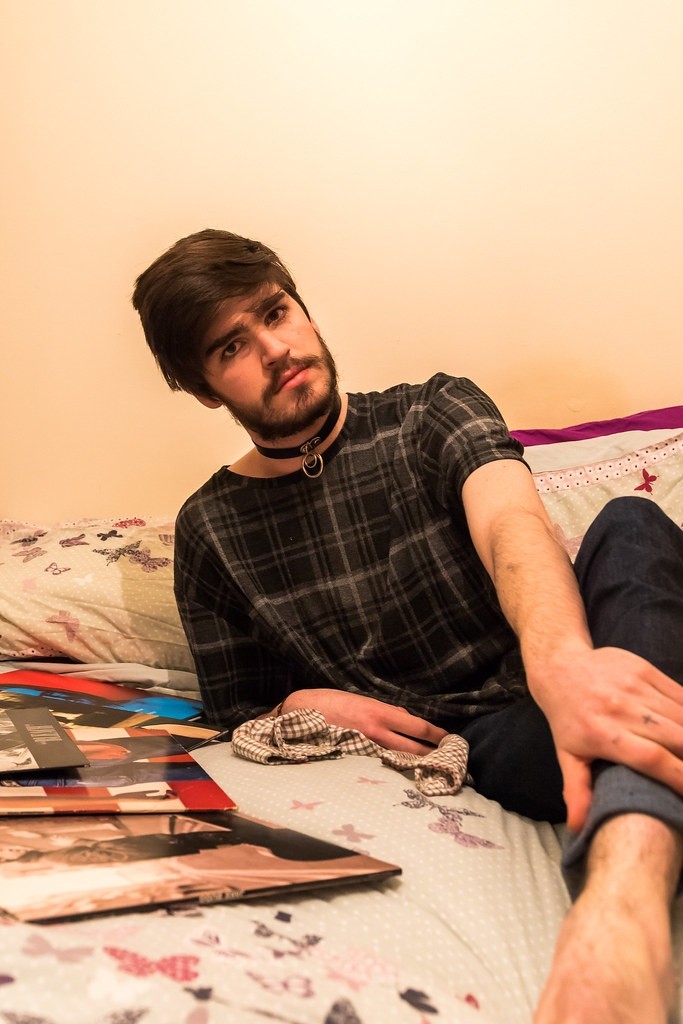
252,391,342,478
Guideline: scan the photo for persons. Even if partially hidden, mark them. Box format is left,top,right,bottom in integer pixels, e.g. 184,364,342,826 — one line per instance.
133,228,683,1024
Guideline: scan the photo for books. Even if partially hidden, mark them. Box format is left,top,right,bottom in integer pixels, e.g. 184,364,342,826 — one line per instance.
0,668,401,926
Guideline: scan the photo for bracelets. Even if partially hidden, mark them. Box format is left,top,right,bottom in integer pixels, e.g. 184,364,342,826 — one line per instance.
277,698,286,717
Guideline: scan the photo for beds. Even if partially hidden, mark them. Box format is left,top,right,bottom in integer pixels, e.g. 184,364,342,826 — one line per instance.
0,404,683,1024
